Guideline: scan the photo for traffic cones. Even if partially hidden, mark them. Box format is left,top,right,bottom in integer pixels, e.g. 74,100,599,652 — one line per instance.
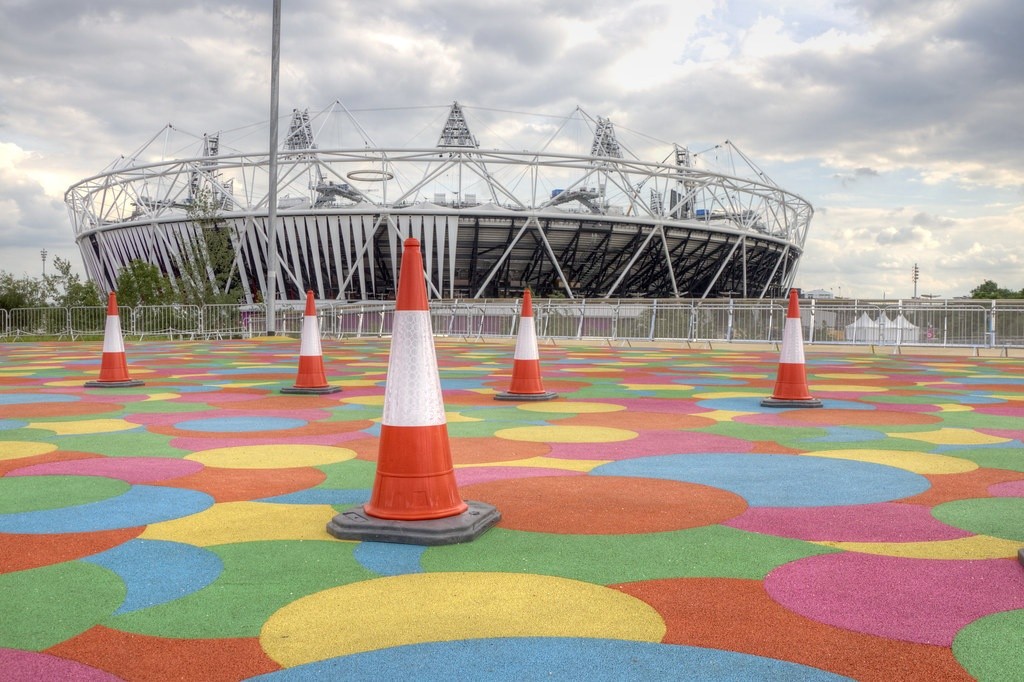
278,289,343,394
492,287,558,401
83,291,145,387
325,237,503,545
759,287,825,408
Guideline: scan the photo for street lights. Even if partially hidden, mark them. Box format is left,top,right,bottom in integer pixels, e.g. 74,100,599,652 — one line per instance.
40,247,48,291
911,262,921,298
